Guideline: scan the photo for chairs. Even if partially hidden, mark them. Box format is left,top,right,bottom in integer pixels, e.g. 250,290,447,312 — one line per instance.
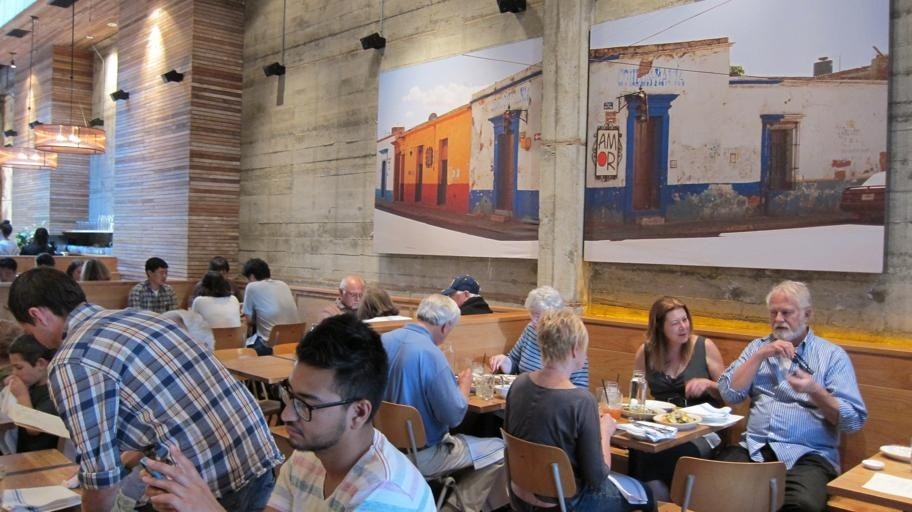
267,322,307,349
499,427,577,512
373,400,467,512
658,456,787,511
213,349,281,430
212,321,249,348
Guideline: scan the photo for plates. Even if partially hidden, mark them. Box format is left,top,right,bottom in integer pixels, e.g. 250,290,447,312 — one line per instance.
703,412,731,422
879,443,911,462
861,457,884,470
625,429,647,440
646,399,675,410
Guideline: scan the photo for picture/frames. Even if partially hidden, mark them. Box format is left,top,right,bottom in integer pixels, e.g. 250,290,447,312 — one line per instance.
374,1,892,273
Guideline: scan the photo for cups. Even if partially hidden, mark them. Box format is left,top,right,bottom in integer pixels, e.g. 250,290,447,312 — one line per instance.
472,362,485,375
600,391,623,422
475,375,495,400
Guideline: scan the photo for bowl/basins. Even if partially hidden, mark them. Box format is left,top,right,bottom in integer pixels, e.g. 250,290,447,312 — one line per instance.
653,411,702,432
496,385,510,398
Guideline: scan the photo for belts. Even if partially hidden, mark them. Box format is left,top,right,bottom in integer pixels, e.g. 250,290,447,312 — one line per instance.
396,447,427,457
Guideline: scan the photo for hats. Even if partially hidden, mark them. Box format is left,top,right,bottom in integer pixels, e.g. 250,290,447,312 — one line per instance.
440,274,480,297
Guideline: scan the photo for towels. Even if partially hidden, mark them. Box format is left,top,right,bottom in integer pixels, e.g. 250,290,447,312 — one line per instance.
0,382,19,453
692,432,722,456
2,484,80,512
460,431,504,471
607,470,649,506
618,420,677,445
247,332,258,345
680,401,732,423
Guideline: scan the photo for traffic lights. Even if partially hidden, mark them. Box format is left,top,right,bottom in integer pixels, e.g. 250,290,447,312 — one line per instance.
632,90,650,124
502,108,528,137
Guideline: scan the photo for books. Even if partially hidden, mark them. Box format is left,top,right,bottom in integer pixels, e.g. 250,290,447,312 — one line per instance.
10,402,71,439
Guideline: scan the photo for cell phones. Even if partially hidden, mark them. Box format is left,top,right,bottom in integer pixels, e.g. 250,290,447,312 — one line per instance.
141,455,168,480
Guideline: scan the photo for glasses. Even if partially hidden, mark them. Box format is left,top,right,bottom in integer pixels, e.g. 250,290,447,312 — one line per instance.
792,352,815,377
277,379,366,422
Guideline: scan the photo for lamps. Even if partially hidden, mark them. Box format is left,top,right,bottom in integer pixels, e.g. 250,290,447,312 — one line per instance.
34,0,106,157
161,70,184,83
360,32,386,50
28,120,43,129
2,129,18,137
263,62,285,78
497,0,527,14
110,89,129,101
9,52,18,67
0,14,57,170
88,118,103,127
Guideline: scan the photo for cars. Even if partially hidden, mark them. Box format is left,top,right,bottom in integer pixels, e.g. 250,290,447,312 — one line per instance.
839,172,886,221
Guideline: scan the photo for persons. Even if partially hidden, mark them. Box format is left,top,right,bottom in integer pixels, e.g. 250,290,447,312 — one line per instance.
0,257,19,283
80,259,111,281
716,280,868,512
379,292,510,511
489,285,589,390
314,276,367,322
627,296,729,480
36,253,57,269
66,259,85,281
0,220,19,254
140,312,437,512
6,329,58,451
20,228,53,255
505,306,669,512
444,274,494,314
127,257,180,312
7,266,285,512
192,271,242,328
242,258,303,354
354,286,414,321
187,256,244,305
